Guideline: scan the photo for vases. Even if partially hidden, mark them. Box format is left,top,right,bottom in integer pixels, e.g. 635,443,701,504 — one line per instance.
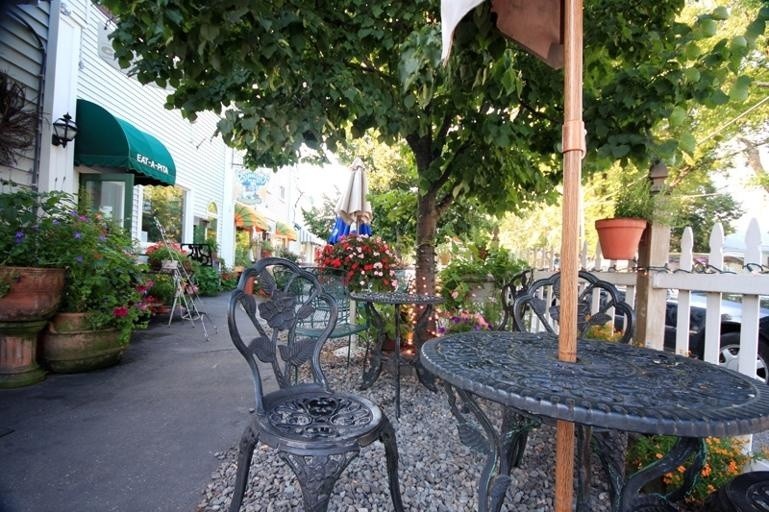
2,258,133,391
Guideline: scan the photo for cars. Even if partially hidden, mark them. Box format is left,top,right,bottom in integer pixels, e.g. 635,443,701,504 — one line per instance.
600,288,769,383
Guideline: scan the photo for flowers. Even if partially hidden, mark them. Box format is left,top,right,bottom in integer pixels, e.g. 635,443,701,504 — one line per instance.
314,234,401,293
0,186,157,345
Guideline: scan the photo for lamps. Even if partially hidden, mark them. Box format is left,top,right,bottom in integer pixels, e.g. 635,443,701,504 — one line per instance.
51,111,80,148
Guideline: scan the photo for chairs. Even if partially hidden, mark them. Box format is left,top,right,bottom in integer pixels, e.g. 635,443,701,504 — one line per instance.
502,268,648,505
224,255,410,512
497,267,536,334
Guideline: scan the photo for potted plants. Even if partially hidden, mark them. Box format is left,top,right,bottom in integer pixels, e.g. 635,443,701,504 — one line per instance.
594,180,687,262
361,299,410,350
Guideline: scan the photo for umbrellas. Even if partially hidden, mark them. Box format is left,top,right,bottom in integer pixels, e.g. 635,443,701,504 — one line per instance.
327,158,373,351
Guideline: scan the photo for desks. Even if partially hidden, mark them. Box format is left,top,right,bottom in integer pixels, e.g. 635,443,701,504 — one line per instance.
349,286,445,399
421,326,762,512
